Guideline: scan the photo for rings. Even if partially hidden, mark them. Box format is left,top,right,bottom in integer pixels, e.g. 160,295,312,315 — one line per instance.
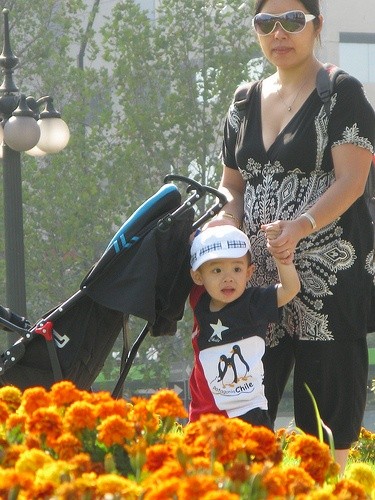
287,249,290,254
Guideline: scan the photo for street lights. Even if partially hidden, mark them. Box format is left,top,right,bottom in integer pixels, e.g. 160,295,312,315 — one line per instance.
0,8,68,353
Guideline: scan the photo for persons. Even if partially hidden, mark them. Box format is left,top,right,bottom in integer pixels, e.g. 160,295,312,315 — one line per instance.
190,224,301,429
199,0,375,480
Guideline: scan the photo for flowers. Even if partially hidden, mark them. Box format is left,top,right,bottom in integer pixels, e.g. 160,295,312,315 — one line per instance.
0,381,375,500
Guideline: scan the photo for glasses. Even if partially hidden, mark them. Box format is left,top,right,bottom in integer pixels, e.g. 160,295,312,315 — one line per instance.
251,9,316,36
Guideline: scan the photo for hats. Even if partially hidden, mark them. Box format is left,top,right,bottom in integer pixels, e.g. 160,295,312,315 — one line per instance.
189,224,252,272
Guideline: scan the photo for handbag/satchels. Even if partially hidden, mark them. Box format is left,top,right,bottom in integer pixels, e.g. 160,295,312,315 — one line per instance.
316,62,375,227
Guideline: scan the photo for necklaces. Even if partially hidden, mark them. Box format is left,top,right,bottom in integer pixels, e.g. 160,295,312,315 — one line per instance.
271,66,309,112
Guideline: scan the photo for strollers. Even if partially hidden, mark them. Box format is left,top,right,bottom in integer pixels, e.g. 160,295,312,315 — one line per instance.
0,173,229,400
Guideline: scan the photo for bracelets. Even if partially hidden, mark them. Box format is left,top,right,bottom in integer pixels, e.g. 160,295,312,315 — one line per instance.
301,213,316,231
213,212,240,229
